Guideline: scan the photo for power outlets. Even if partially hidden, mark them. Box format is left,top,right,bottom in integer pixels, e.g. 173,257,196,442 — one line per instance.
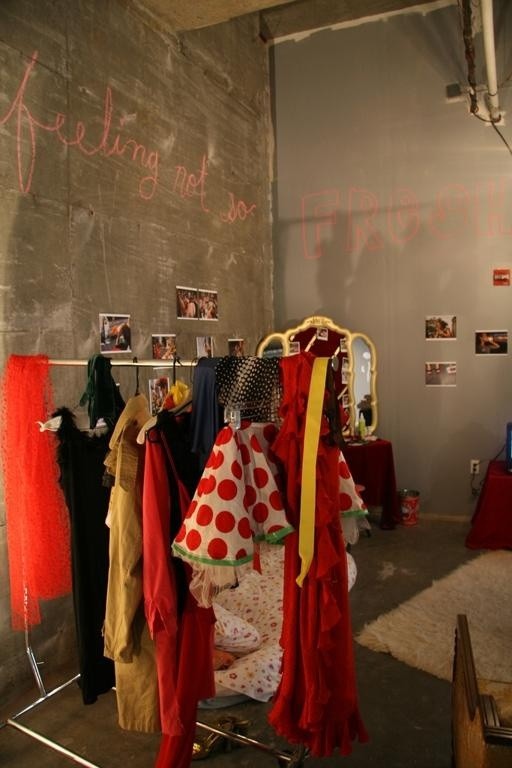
470,460,479,474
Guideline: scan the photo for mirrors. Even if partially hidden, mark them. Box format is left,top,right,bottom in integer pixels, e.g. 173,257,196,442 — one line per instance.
346,333,377,438
256,333,288,357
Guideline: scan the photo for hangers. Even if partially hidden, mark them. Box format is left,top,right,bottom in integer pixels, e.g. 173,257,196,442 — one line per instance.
132,356,141,396
190,357,199,385
173,356,179,384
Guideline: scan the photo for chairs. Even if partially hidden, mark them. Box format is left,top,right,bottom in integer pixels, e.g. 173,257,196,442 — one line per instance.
452,615,512,768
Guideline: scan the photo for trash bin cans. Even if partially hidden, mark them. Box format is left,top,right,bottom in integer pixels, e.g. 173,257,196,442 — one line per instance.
398,488,420,526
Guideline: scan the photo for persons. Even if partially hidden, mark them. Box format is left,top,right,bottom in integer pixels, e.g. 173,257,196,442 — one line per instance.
434,320,451,338
178,292,218,319
479,334,500,353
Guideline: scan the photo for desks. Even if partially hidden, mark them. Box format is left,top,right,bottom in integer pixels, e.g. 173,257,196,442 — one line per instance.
339,437,401,530
465,459,512,549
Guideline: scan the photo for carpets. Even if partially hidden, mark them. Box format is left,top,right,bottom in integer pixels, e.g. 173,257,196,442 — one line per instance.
355,550,512,728
